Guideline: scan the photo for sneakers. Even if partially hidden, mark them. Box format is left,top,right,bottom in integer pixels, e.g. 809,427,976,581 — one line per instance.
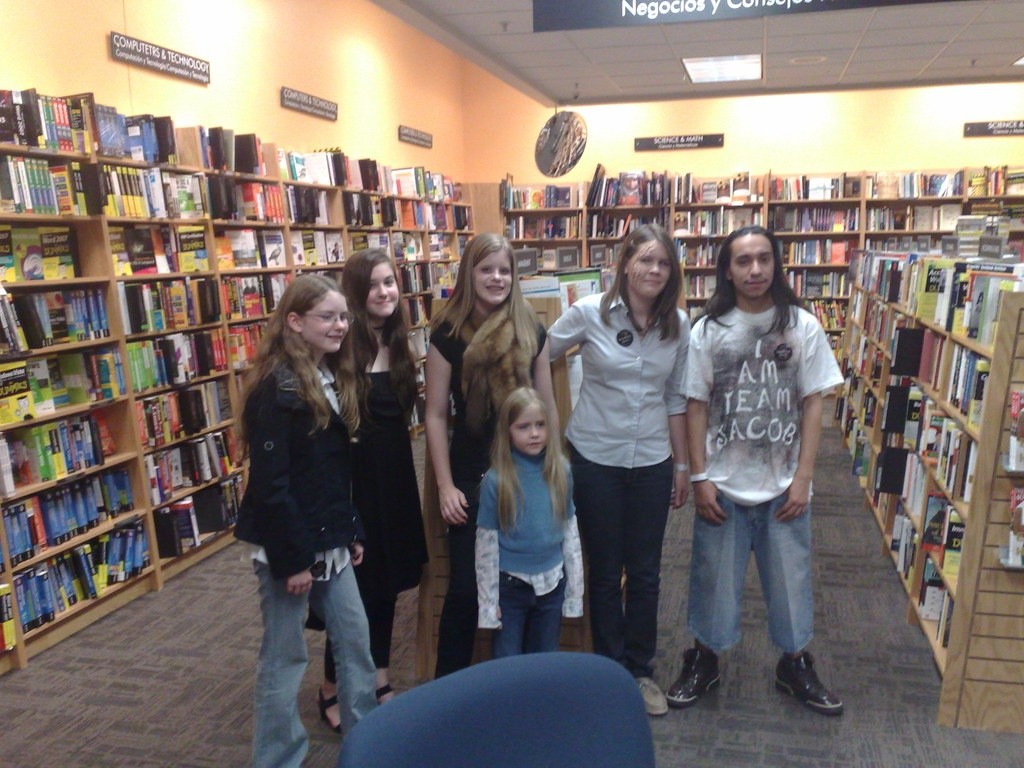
667,648,720,702
776,651,843,708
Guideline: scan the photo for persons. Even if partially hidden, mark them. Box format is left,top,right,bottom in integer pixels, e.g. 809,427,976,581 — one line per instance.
232,274,378,768
546,223,691,715
424,233,561,681
474,388,584,660
318,248,431,735
665,224,845,715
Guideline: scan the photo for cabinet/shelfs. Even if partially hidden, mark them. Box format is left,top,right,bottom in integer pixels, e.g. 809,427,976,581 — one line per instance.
0,142,1024,732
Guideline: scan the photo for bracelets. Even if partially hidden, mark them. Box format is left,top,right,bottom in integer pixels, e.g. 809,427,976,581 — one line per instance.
690,473,707,482
673,463,688,472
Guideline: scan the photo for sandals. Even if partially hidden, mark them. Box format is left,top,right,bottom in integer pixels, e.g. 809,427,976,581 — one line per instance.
376,683,396,704
319,687,342,734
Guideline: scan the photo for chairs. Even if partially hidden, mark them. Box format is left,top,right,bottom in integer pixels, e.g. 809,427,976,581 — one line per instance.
338,651,656,768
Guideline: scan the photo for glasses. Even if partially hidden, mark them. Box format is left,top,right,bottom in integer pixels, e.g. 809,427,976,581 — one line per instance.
304,311,355,324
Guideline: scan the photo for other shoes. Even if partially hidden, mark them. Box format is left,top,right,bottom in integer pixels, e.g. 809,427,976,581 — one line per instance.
638,678,669,715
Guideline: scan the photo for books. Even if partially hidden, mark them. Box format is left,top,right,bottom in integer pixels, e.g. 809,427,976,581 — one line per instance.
500,163,1024,358
0,89,474,652
1009,390,1024,470
1008,487,1024,566
832,289,991,647
